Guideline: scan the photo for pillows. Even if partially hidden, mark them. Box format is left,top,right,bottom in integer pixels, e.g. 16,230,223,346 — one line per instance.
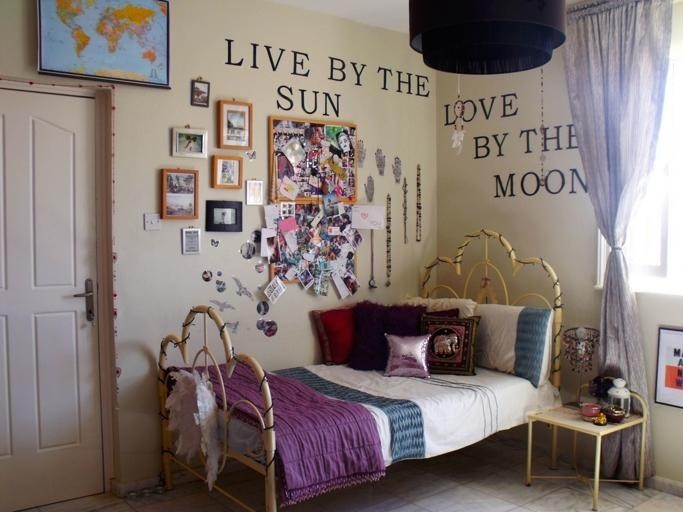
348,300,428,370
474,304,554,388
385,333,432,379
420,314,482,376
402,296,477,319
312,306,353,366
424,309,459,317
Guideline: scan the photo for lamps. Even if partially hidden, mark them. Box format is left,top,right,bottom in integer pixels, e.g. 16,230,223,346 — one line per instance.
563,327,600,410
409,0,567,186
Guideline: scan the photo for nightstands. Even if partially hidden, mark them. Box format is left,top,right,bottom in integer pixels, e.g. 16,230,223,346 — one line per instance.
526,383,648,511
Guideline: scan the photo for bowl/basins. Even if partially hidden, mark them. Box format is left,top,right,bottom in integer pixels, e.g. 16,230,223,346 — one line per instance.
607,406,626,423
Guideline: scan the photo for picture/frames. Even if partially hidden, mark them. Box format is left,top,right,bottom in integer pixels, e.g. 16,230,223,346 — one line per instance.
212,154,243,189
37,0,170,88
269,262,300,284
191,79,210,108
172,127,208,159
161,169,199,219
654,327,683,408
268,114,358,204
205,200,243,232
182,228,200,255
218,99,253,150
245,180,263,206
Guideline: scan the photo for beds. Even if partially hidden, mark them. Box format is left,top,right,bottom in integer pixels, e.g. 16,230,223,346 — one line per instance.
157,228,562,512
